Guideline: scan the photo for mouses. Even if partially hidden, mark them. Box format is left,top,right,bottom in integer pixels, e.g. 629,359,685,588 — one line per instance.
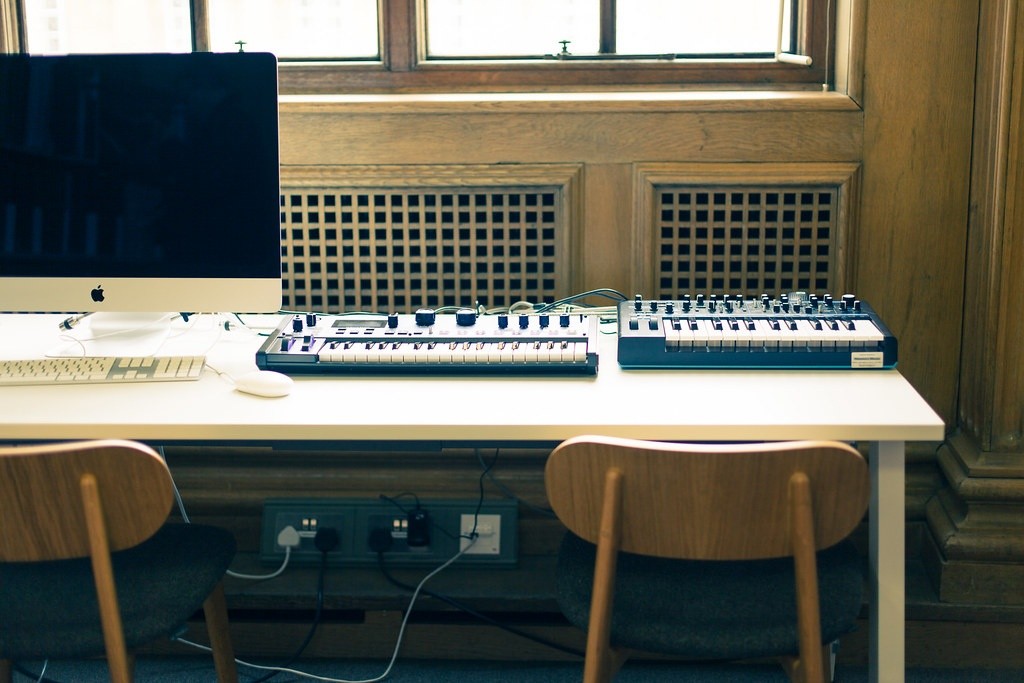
234,370,293,398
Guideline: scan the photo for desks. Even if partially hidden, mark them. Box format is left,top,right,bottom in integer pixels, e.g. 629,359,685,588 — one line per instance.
0,315,944,682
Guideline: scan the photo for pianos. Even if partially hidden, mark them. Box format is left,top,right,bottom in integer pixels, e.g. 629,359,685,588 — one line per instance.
254,309,599,377
616,292,898,371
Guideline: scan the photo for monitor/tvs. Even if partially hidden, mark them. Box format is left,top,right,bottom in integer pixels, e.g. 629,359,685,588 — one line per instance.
0,50,283,314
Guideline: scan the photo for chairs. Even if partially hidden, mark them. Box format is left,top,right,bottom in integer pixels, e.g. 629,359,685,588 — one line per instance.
0,440,240,683
546,435,869,682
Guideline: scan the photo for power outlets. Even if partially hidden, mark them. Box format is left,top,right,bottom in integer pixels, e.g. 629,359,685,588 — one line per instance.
275,510,353,555
368,515,429,552
459,513,501,554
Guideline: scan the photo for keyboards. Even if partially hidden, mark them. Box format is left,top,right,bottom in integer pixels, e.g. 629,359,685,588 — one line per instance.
0,355,207,382
